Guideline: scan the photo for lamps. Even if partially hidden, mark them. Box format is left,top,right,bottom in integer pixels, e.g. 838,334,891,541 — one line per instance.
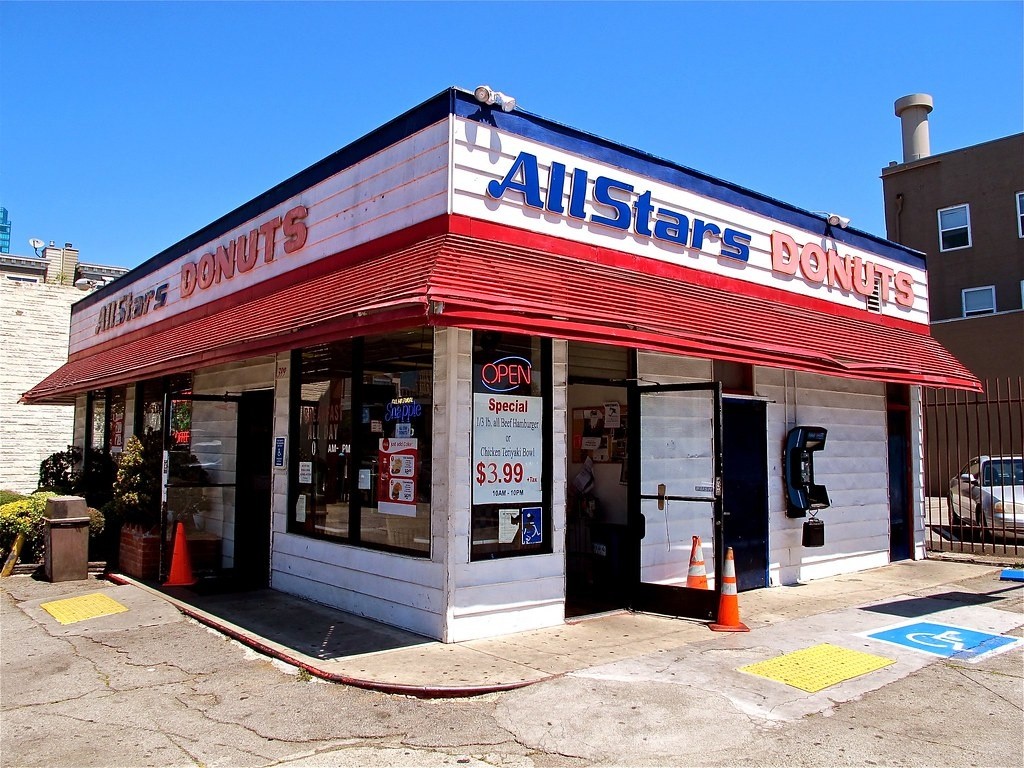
810,210,852,229
474,84,517,112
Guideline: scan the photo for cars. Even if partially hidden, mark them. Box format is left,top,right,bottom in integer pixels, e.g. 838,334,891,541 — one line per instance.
951,455,1024,546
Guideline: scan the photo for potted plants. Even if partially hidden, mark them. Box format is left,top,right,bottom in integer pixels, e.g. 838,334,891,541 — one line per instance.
113,427,223,580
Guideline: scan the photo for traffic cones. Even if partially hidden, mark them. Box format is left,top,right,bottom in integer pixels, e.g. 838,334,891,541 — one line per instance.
686,536,709,591
707,546,751,632
163,522,201,586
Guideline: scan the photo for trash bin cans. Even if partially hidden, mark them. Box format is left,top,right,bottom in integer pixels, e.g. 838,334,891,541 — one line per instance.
43,496,90,582
